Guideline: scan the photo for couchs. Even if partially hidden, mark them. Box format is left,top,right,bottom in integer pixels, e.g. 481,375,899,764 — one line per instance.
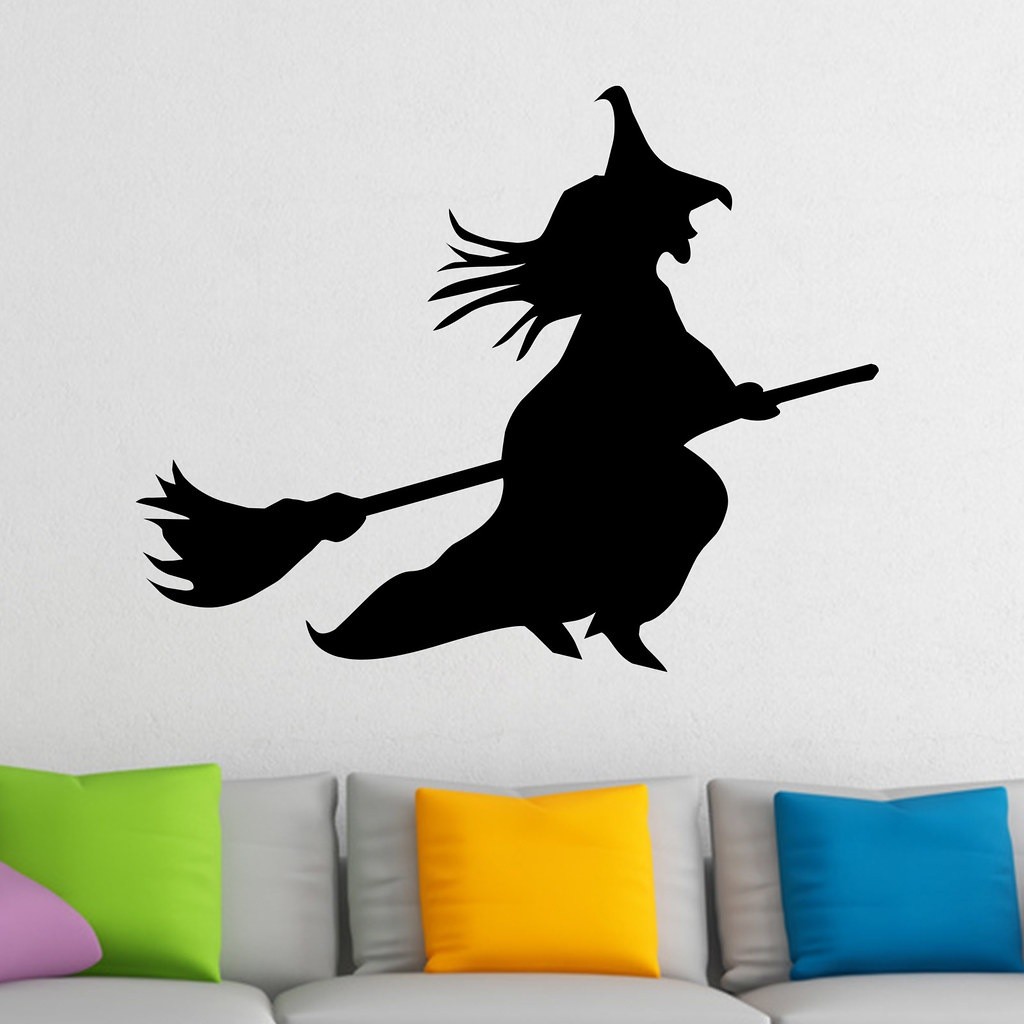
0,762,1024,1024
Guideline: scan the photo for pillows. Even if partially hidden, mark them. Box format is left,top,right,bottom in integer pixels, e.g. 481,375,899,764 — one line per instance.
706,777,1024,994
773,786,1024,981
0,863,104,986
415,783,660,979
346,770,709,984
0,762,222,983
220,771,342,986
